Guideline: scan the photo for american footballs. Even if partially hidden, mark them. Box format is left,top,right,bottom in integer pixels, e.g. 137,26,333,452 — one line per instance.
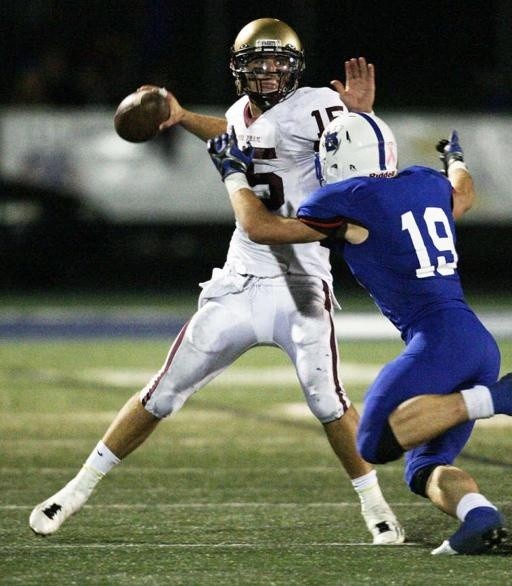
114,91,169,142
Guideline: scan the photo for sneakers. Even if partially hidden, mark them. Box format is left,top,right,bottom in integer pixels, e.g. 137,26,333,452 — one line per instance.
430,508,509,557
28,477,88,537
365,510,406,546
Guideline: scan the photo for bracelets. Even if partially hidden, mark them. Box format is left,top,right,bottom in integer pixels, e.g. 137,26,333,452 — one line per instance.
222,170,253,196
446,161,470,177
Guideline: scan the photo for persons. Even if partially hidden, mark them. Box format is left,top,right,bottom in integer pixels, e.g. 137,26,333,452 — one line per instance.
25,15,409,547
205,123,511,556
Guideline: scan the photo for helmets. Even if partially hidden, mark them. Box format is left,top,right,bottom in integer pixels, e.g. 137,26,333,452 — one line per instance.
228,16,307,107
315,111,398,188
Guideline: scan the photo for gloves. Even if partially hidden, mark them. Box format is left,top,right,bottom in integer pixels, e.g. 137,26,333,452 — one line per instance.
435,129,469,174
206,125,253,197
